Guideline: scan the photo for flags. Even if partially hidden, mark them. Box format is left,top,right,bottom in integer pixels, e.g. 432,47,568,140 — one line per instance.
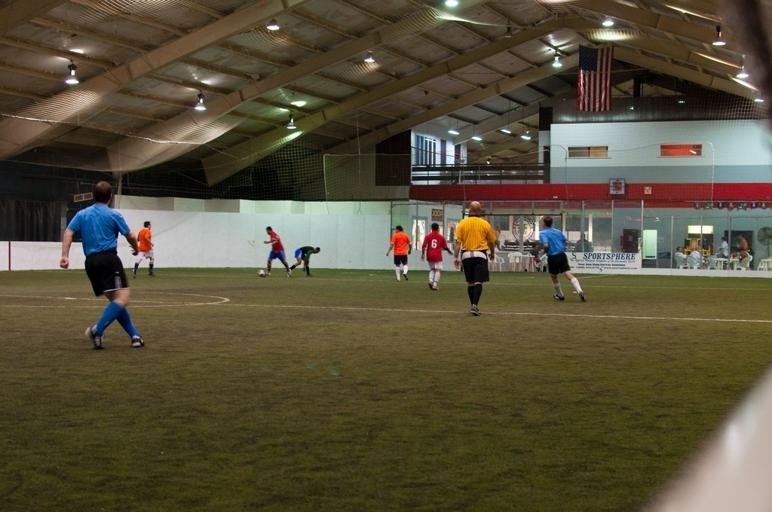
577,43,613,113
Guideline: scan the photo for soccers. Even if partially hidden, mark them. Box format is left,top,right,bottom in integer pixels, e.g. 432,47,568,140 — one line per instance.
258,269,266,277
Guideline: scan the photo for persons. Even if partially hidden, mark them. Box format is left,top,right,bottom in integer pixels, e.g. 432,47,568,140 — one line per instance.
573,232,593,253
453,200,497,316
288,246,320,277
539,216,587,302
264,226,292,278
674,233,749,270
421,223,453,291
132,221,155,278
60,180,144,350
529,244,542,272
386,226,412,282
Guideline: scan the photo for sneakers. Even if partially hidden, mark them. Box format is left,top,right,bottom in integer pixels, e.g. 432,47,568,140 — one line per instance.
552,293,565,301
130,334,144,348
131,269,154,276
578,291,585,302
469,303,480,316
266,269,312,278
395,273,408,282
83,326,105,350
428,282,439,290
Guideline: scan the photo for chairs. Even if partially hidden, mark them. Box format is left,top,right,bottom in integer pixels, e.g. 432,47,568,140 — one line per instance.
530,250,549,273
672,245,772,271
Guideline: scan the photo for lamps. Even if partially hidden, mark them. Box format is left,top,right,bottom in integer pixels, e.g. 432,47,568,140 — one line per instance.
194,89,208,111
265,18,280,30
734,54,748,80
444,0,458,8
678,89,687,105
290,97,307,107
63,64,79,85
503,17,512,38
753,91,765,104
363,52,375,64
447,128,532,142
601,17,615,27
285,110,296,130
711,25,726,47
551,49,562,68
